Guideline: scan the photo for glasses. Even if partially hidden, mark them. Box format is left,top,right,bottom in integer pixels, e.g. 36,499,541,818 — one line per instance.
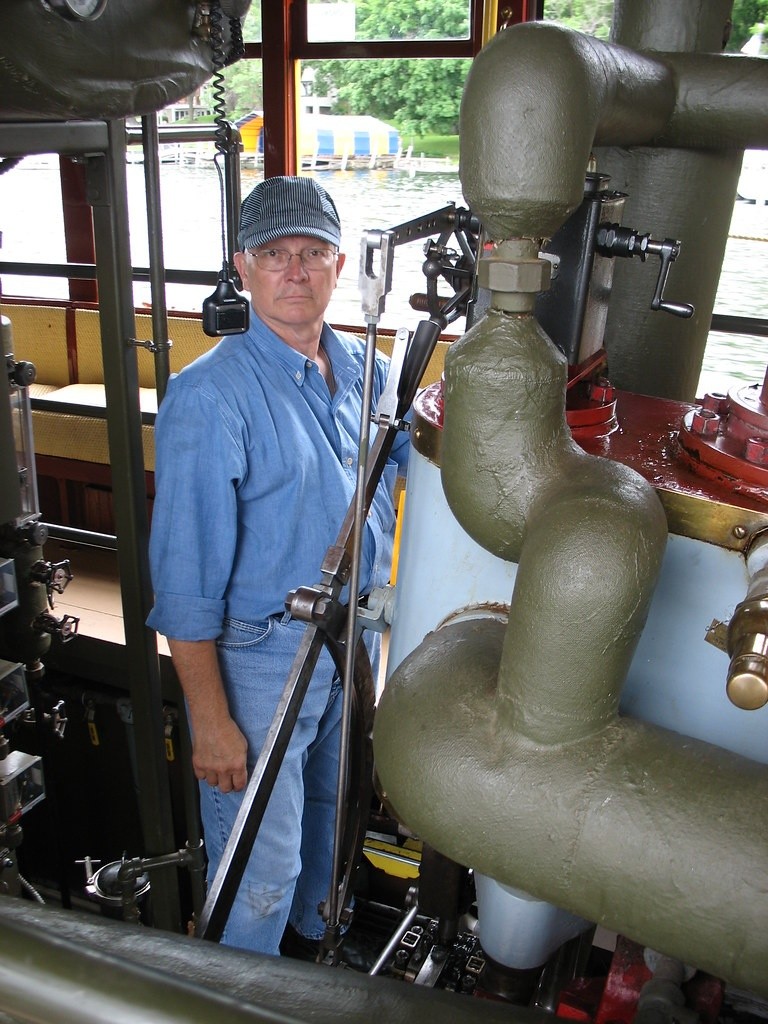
244,249,337,272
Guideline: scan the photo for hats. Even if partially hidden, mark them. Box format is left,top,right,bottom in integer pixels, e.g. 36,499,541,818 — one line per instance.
237,176,342,252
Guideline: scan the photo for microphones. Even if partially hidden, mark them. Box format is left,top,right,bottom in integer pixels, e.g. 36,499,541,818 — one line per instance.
202,277,249,338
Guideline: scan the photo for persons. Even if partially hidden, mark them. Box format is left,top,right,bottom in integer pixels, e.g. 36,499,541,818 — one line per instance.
142,176,411,956
